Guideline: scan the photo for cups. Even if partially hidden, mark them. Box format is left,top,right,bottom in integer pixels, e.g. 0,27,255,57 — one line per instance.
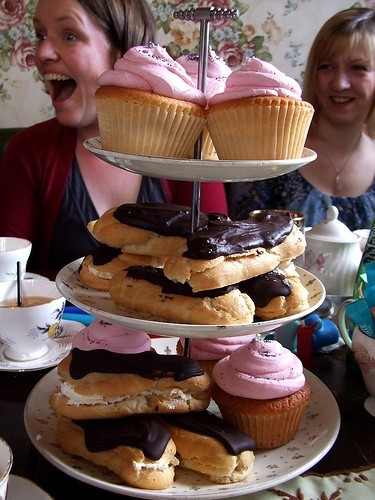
353,229,372,251
0,279,66,361
0,437,13,500
0,237,32,279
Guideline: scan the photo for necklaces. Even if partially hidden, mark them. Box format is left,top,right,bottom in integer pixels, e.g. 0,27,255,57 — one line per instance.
318,130,362,195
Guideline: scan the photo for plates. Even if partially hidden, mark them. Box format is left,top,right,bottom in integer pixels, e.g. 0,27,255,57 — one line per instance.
0,320,89,372
24,272,49,280
7,474,54,500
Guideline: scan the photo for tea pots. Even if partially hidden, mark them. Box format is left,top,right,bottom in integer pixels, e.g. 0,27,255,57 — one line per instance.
303,205,362,296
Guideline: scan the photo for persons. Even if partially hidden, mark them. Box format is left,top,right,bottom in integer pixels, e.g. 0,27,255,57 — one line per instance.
0,0,228,281
223,6,375,262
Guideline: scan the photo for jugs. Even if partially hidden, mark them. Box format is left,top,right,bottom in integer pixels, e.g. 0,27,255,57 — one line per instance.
337,300,375,417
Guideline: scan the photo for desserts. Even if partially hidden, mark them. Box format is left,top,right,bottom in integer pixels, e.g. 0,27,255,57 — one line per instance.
77,202,309,326
94,40,208,159
205,55,315,160
49,319,259,490
174,45,232,160
210,339,311,450
